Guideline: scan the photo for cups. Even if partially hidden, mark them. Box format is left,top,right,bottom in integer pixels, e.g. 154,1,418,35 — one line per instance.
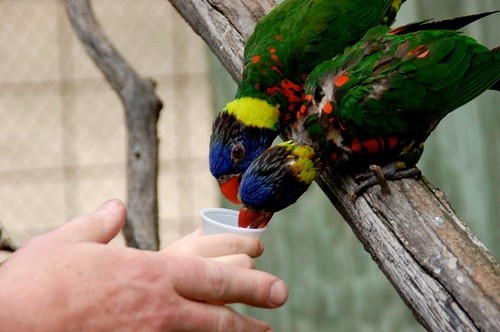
201,209,266,240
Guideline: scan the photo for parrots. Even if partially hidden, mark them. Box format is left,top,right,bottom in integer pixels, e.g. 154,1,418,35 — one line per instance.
237,8,500,229
209,0,407,207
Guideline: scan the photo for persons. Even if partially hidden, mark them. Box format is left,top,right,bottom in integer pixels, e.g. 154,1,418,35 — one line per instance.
0,198,290,332
